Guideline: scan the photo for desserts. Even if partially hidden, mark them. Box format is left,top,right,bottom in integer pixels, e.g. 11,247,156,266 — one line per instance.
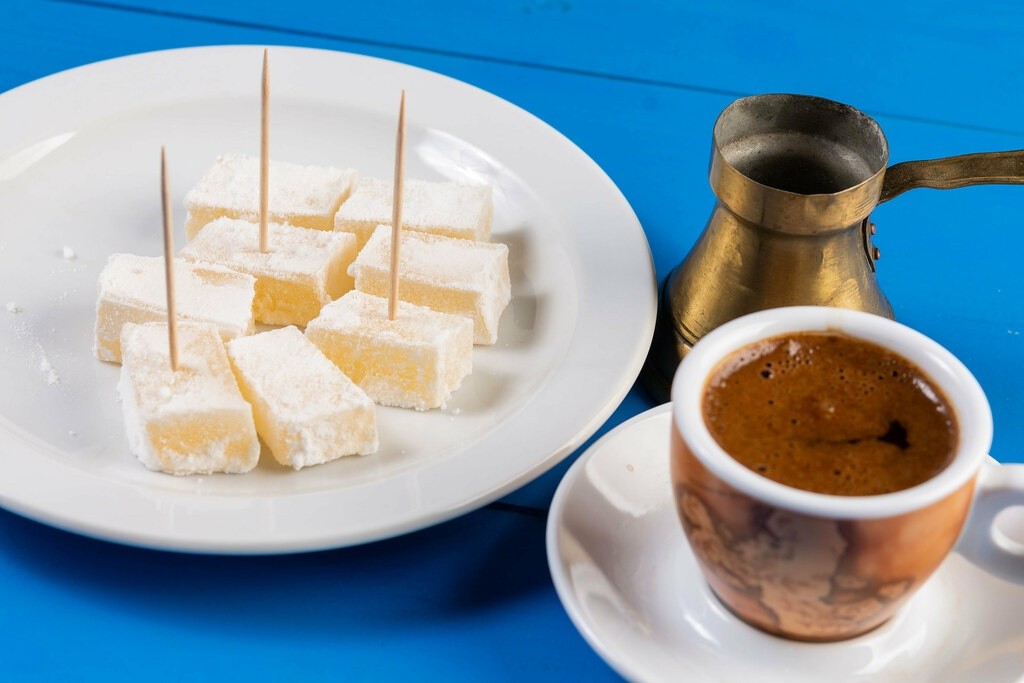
93,155,511,474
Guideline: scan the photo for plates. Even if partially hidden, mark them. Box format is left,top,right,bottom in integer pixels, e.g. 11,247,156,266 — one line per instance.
544,403,1024,683
0,43,658,556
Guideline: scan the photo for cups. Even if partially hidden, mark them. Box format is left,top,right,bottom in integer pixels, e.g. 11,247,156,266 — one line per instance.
670,303,993,639
